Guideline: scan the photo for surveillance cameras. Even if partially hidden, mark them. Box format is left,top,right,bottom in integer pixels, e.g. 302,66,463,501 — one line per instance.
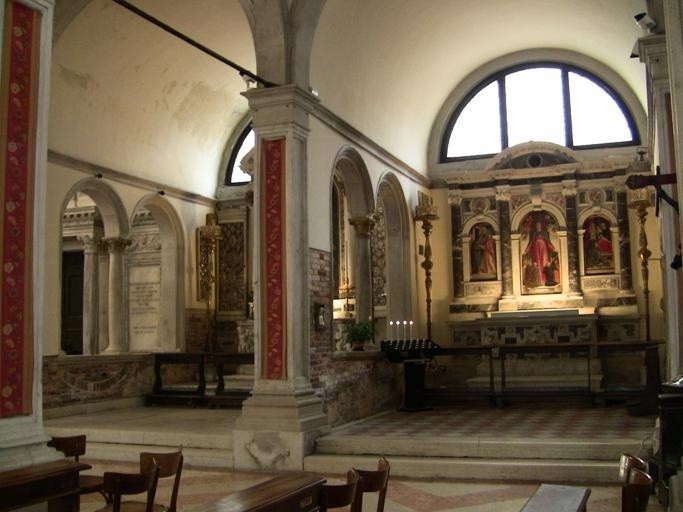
240,70,257,85
634,11,657,37
629,39,641,59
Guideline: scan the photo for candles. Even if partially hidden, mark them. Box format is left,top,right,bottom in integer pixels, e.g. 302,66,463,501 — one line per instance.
387,320,413,340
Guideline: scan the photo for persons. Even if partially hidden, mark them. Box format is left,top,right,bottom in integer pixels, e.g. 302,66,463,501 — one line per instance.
521,213,561,287
472,224,497,279
596,221,613,252
601,221,611,240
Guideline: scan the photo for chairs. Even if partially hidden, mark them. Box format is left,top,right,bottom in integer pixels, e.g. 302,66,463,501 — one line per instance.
49,432,108,506
101,458,159,511
352,456,396,512
120,443,183,512
319,469,358,512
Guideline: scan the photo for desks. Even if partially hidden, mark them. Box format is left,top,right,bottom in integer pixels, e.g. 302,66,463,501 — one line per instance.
424,340,647,410
0,458,90,511
145,350,211,394
210,352,254,396
185,470,328,512
